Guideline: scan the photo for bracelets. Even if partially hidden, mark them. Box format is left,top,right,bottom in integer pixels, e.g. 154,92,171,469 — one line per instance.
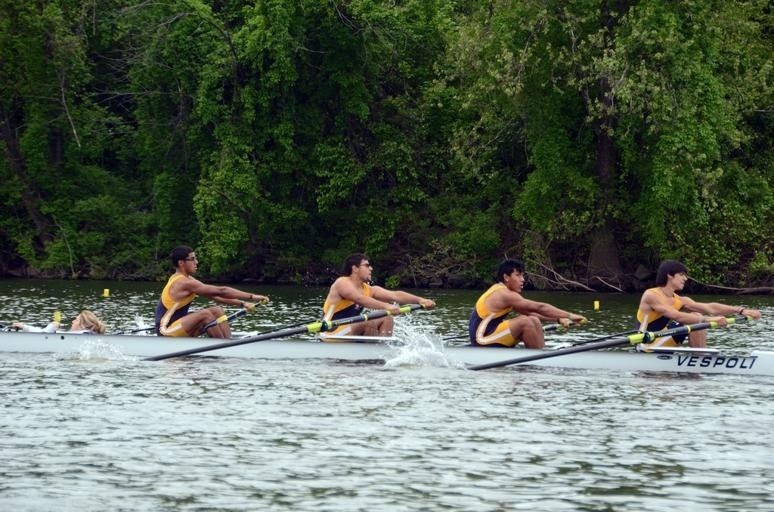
241,301,244,306
739,309,744,315
557,317,560,324
250,293,253,300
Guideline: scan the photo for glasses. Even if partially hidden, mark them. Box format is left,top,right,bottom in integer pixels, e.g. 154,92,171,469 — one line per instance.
358,263,370,268
183,256,197,262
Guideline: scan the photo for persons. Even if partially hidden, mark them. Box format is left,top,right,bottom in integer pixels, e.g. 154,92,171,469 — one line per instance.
155,245,269,339
469,260,588,350
317,253,436,344
12,310,105,336
632,259,762,357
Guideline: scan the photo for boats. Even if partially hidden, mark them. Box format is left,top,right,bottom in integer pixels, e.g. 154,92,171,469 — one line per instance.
1,322,773,379
